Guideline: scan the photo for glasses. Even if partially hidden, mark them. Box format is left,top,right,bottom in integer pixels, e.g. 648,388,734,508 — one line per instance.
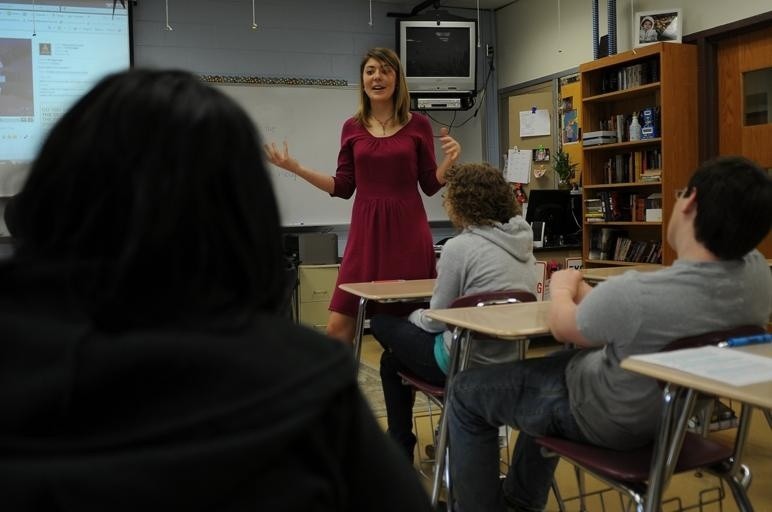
673,187,690,199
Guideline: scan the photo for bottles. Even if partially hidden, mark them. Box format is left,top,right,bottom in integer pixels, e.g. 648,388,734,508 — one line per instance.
629,116,641,141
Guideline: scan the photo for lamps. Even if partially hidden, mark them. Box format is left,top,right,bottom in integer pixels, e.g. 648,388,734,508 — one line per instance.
554,0,564,53
248,1,258,29
161,0,175,33
476,0,483,48
367,1,375,31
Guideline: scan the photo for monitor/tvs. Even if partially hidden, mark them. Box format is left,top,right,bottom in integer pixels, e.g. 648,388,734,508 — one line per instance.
525,189,574,247
395,17,478,98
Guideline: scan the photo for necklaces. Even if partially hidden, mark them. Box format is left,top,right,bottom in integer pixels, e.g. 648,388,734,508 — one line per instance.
371,114,395,136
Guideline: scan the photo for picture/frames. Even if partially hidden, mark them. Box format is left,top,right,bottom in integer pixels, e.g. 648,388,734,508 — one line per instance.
635,8,681,46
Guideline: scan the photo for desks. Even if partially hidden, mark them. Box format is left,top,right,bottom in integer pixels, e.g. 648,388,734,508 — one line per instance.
520,242,579,280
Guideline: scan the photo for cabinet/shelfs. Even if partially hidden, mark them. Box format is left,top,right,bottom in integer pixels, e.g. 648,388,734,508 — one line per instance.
286,262,339,335
580,43,702,271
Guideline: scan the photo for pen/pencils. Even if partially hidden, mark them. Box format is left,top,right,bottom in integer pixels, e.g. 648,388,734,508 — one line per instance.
716,333,772,349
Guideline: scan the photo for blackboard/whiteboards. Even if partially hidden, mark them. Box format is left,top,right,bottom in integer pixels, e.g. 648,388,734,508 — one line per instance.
198,74,490,235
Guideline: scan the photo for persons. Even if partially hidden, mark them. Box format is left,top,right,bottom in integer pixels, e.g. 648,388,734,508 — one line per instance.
445,152,772,512
638,15,659,43
0,66,435,511
368,161,538,469
261,46,463,348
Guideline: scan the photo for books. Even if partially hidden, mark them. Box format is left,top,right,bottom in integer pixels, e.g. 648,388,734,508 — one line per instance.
578,57,664,268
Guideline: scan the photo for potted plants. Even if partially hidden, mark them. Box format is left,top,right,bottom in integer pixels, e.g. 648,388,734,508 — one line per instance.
552,147,579,189
